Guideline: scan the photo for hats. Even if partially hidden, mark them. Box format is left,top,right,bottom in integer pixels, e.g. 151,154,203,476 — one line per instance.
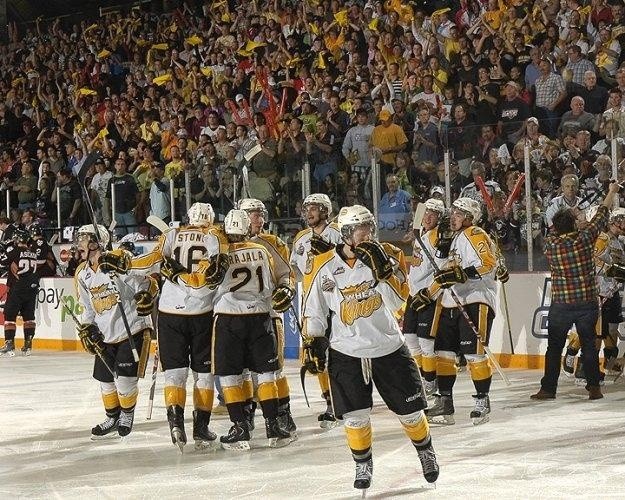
177,129,187,136
95,159,105,164
505,81,517,88
236,94,244,103
379,110,392,121
528,116,538,126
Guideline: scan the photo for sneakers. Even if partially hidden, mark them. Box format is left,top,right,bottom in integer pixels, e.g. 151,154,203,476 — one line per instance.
355,458,372,488
92,412,134,436
470,396,490,417
564,347,620,378
0,341,32,352
589,386,602,400
319,405,336,421
171,413,296,443
530,389,555,399
418,446,439,482
423,378,454,415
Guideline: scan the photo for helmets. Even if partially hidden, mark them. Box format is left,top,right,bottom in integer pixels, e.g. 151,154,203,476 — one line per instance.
338,205,376,237
224,199,268,234
302,193,332,216
14,227,41,244
426,197,481,225
78,224,110,250
188,202,214,227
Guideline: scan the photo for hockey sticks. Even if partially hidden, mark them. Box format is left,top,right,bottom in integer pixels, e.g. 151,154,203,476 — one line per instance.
144,213,171,421
299,366,320,409
58,294,119,379
232,143,263,209
77,147,141,363
411,202,513,388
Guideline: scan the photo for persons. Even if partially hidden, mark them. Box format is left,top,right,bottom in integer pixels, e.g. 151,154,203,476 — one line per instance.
302,204,440,489
1,2,623,453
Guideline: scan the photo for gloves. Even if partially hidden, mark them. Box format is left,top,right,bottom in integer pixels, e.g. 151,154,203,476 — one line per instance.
303,336,330,374
79,323,105,354
161,253,231,289
412,267,467,312
496,265,509,283
99,253,126,275
309,236,334,255
133,290,153,316
272,287,295,312
356,241,392,279
